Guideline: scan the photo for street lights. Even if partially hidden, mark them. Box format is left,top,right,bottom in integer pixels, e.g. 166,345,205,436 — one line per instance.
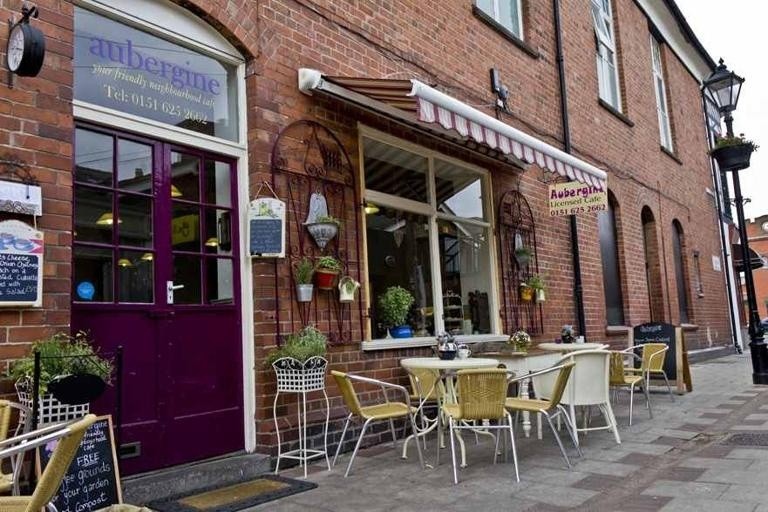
702,57,767,385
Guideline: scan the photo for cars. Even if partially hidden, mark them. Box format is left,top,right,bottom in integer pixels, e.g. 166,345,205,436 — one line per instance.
747,318,767,335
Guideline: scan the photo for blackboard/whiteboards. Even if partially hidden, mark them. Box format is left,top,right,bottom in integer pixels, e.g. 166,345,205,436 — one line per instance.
628,322,683,386
248,217,285,257
36,415,125,512
0,253,43,307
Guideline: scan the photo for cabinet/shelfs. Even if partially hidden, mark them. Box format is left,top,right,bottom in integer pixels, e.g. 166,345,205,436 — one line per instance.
443,289,464,336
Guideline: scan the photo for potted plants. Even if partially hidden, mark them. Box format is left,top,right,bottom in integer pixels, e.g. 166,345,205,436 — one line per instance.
561,324,574,343
302,216,342,251
35,333,113,405
294,257,314,303
6,345,90,424
513,247,532,270
530,276,545,304
338,276,360,304
509,330,532,352
315,256,340,290
706,131,760,171
377,285,411,337
264,324,328,393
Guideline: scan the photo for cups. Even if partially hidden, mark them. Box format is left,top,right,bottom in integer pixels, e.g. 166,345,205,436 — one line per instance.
575,339,582,345
554,338,561,344
458,349,471,360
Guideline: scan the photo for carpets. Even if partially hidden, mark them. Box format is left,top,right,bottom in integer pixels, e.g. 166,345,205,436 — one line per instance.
152,476,319,512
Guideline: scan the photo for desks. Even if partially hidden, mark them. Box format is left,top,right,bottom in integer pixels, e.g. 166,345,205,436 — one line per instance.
400,356,500,470
536,341,604,436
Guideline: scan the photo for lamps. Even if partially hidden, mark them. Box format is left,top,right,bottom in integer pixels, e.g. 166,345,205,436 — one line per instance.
204,238,219,247
365,203,380,215
171,185,183,197
118,258,132,267
95,213,122,225
140,253,154,260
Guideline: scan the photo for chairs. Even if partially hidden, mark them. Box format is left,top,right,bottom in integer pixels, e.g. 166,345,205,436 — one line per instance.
622,343,675,409
0,412,96,511
404,368,449,451
434,367,521,485
528,348,622,446
330,370,428,478
608,349,652,427
492,362,583,469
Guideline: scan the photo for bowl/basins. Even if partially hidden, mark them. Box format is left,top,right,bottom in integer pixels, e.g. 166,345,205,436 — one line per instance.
0,233,32,251
437,350,456,360
